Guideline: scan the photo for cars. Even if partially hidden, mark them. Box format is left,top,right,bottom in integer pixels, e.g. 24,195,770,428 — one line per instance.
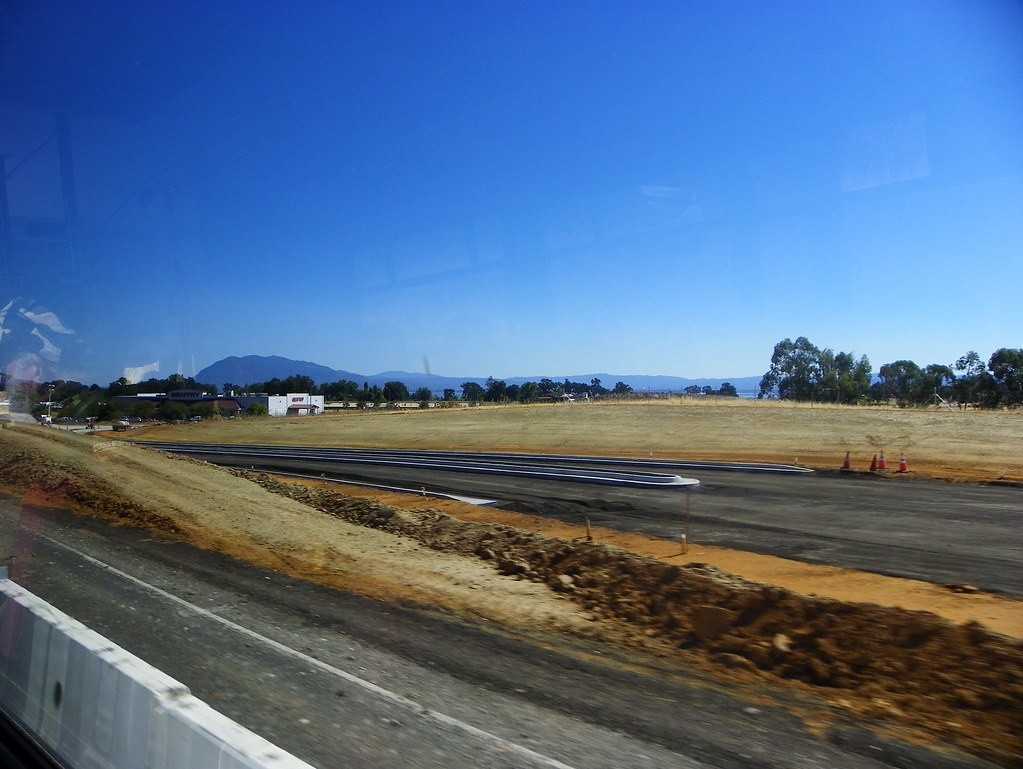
113,415,143,431
0,400,13,405
56,415,92,422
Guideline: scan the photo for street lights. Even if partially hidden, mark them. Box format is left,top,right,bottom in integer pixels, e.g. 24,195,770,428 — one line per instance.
877,450,887,471
840,451,851,470
48,385,55,417
867,454,879,472
894,451,912,474
